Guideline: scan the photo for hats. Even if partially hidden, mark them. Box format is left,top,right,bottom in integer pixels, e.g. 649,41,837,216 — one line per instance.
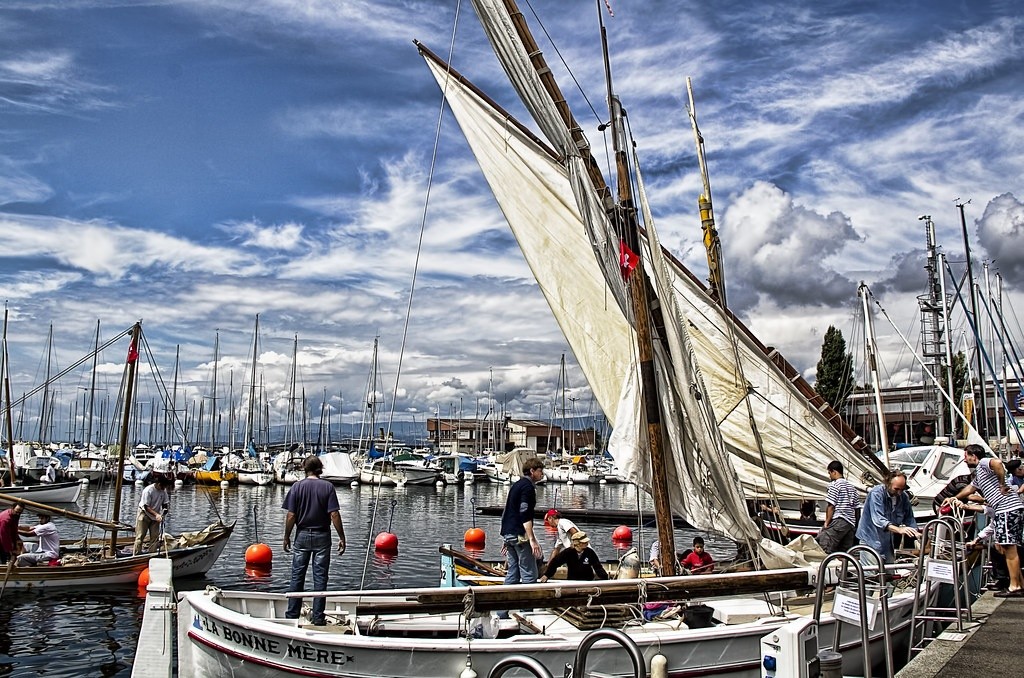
571,531,591,543
544,509,560,520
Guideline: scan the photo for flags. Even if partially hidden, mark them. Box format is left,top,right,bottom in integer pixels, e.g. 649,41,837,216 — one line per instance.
619,240,639,281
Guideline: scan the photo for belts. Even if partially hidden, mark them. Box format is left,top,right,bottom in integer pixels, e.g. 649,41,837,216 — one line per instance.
303,527,329,531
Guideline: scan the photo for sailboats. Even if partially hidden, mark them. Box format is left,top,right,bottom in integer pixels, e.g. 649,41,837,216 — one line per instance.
185,0,1024,678
0,296,239,593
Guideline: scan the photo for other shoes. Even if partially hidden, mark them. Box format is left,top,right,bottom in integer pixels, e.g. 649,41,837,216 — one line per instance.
989,581,1010,591
995,589,1022,597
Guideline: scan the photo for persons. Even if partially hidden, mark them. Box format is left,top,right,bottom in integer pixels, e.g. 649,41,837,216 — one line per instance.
133,477,169,555
681,537,714,575
855,472,919,601
814,461,860,554
650,540,660,569
943,443,1024,598
498,458,546,619
17,513,60,567
0,502,25,564
281,456,346,625
540,531,608,583
547,509,592,565
45,460,57,483
933,470,984,520
